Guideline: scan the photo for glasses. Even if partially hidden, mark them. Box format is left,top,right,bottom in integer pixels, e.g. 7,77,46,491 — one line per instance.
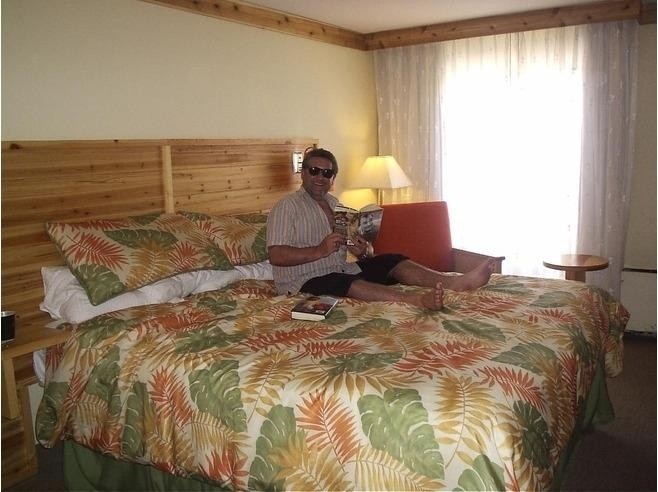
304,164,336,178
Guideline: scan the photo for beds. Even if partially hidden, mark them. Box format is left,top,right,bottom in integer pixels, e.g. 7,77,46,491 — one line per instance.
36,257,620,491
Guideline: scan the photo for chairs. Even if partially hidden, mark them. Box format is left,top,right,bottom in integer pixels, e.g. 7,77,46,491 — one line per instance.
374,200,506,278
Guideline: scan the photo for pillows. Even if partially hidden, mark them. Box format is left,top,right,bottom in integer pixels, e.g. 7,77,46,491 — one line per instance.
37,206,273,326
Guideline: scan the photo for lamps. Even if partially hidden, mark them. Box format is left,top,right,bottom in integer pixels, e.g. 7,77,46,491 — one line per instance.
348,156,413,205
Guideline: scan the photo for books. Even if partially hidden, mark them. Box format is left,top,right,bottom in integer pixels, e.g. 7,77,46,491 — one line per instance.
331,204,384,246
291,296,339,322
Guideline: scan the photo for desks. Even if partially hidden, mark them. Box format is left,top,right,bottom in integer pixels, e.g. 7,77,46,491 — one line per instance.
543,252,611,284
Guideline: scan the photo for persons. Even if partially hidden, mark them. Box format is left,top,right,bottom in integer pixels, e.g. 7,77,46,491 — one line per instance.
265,148,495,311
